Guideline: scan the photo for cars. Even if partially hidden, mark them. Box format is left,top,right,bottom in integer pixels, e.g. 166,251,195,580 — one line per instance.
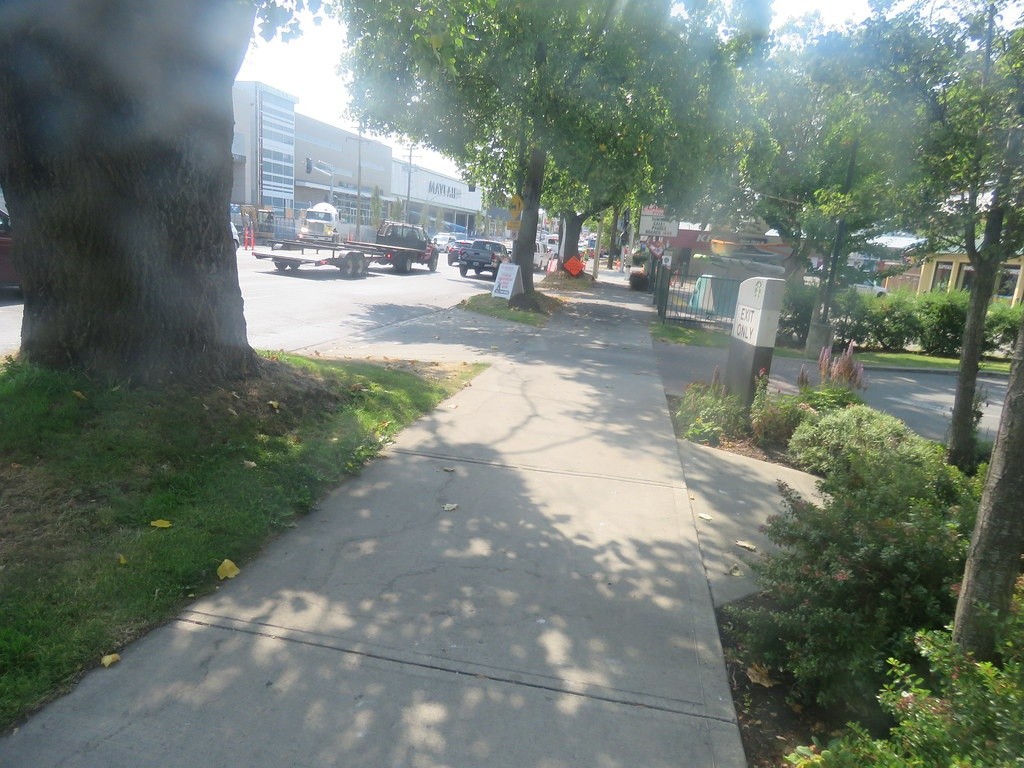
231,223,240,249
0,209,23,298
433,234,458,252
804,263,886,297
447,239,474,266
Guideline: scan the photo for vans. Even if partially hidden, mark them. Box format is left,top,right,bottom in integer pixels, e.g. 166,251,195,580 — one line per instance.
545,234,558,259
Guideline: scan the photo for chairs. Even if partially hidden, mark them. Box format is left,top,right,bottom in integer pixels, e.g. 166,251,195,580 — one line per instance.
323,214,332,220
389,228,399,236
406,231,417,239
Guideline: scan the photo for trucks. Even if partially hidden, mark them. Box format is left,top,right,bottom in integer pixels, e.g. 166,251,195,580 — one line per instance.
252,219,440,278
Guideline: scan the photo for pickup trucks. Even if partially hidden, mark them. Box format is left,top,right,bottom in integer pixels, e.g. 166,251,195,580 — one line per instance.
460,240,513,277
533,241,549,275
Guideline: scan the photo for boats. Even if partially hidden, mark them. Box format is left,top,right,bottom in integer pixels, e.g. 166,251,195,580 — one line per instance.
711,234,793,264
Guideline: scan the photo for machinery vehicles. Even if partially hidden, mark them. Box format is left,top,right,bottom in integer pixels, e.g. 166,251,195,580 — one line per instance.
297,202,342,243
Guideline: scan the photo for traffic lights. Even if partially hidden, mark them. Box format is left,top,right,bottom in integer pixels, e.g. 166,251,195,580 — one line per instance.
306,158,312,174
